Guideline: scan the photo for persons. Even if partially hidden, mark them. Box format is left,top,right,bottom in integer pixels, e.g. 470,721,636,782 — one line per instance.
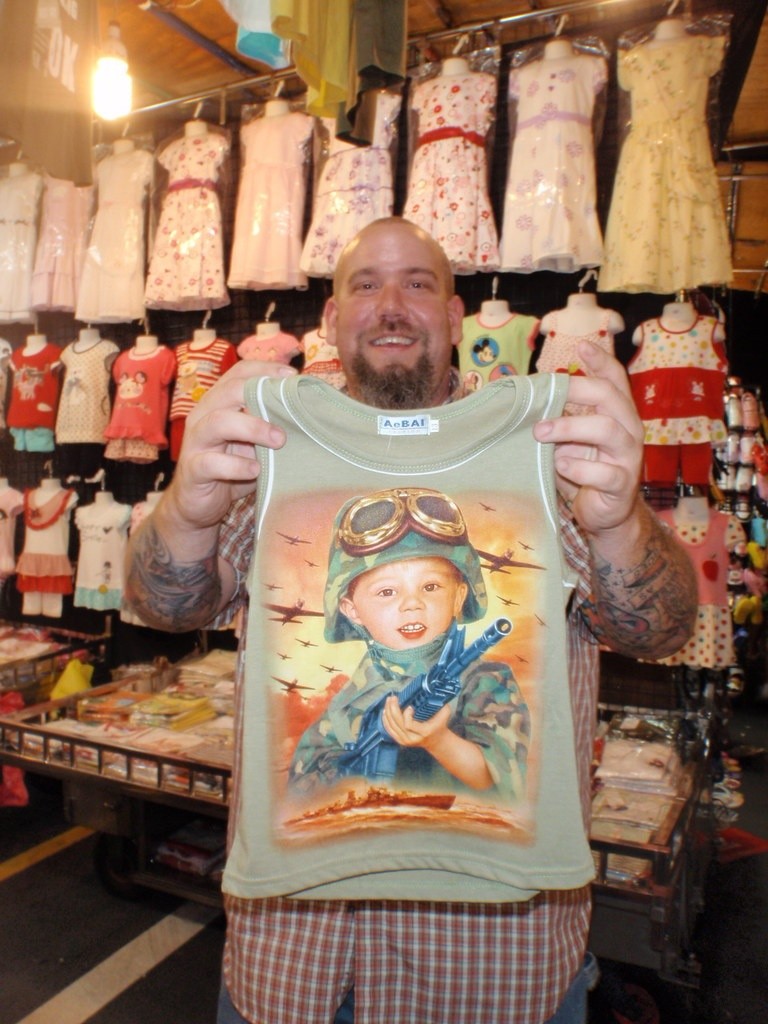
73,492,168,628
597,21,735,293
498,40,608,274
143,121,231,310
227,99,314,291
15,478,78,618
55,328,120,443
0,163,93,325
75,140,155,323
626,302,725,486
103,335,177,464
456,300,540,390
637,496,745,668
6,334,64,451
124,216,699,1024
539,293,625,373
0,477,24,579
403,57,501,275
168,329,238,462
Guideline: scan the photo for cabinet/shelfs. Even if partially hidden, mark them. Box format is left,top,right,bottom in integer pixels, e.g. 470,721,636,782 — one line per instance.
2,618,723,1024
